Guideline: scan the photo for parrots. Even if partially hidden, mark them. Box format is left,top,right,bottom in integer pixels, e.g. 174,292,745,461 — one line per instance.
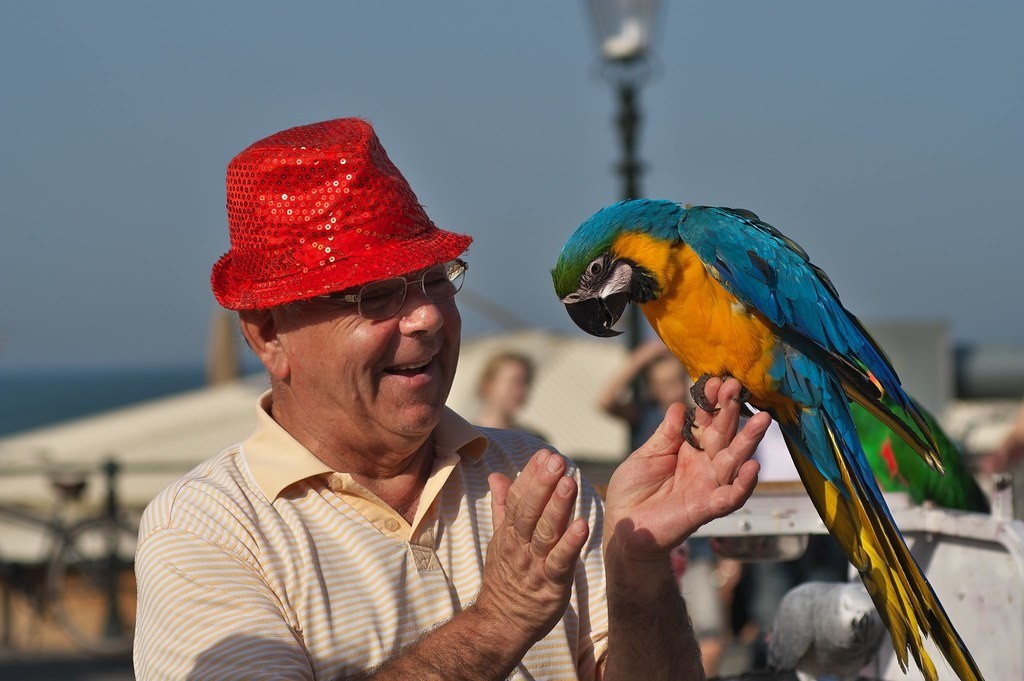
547,194,991,681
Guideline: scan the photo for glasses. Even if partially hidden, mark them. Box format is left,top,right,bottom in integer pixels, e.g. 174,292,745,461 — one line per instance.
312,257,468,320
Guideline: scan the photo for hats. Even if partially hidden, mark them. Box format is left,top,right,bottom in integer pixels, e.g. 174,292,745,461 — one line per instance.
210,117,475,311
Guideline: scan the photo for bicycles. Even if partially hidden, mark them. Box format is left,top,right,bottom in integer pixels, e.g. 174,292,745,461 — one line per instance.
1,481,142,659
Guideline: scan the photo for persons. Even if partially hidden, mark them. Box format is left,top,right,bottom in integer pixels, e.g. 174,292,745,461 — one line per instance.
969,397,1024,480
467,344,554,446
598,339,849,681
132,117,772,681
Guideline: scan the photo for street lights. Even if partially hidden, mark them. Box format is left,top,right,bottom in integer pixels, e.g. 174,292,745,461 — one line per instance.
582,1,666,452
